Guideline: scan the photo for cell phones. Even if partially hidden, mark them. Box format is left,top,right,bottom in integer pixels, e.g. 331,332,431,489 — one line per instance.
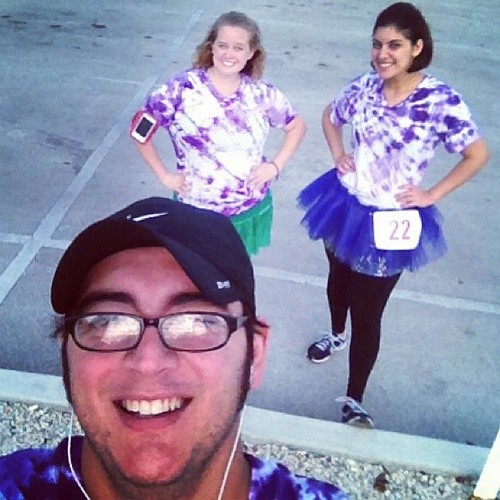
131,113,157,144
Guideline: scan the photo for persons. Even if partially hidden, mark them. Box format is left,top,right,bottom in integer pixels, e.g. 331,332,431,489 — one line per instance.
130,13,308,258
0,197,347,500
293,3,489,428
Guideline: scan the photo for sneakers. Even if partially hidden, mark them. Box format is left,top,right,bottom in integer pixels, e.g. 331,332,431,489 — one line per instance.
334,396,375,429
306,331,347,363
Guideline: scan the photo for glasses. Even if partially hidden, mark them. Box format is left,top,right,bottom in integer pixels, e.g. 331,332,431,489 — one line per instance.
66,312,249,353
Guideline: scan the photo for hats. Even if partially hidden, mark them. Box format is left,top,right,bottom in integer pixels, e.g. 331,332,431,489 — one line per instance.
51,197,256,316
